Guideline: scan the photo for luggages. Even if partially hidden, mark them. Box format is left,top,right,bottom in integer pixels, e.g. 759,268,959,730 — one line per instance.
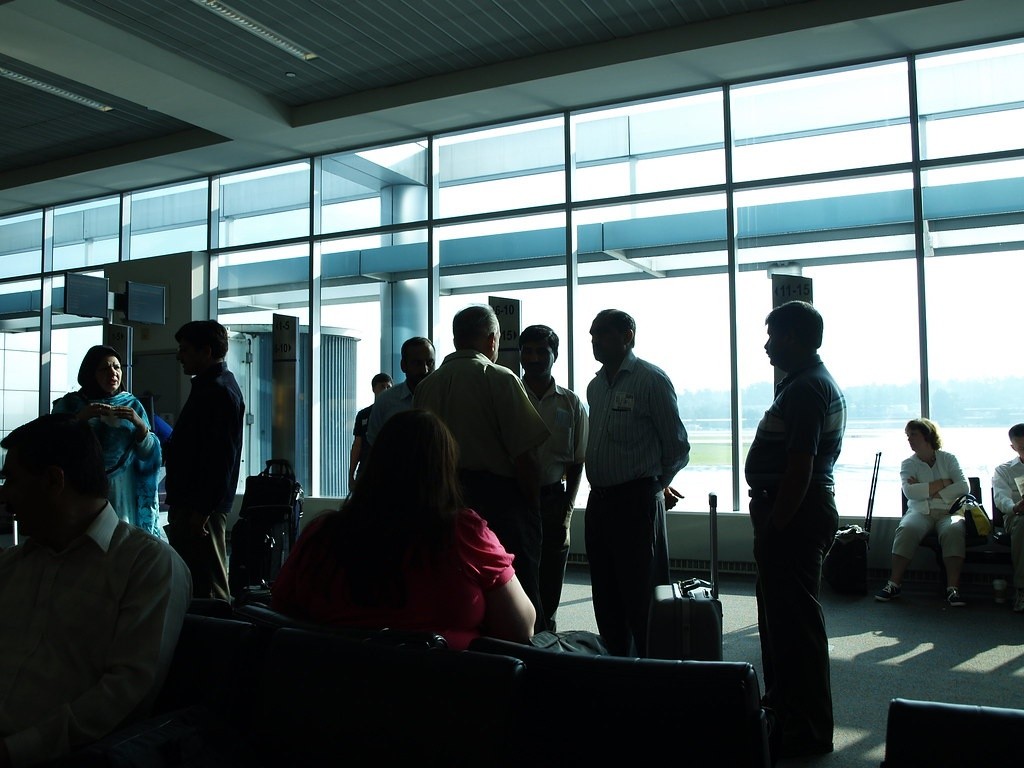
824,447,882,596
230,459,300,591
644,492,726,662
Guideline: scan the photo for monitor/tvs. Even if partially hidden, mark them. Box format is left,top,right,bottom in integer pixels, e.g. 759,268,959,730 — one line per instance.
64,272,110,319
126,281,165,325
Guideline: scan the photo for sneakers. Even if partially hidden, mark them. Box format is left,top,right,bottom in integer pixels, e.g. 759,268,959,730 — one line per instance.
1011,592,1024,612
945,586,964,607
874,582,902,602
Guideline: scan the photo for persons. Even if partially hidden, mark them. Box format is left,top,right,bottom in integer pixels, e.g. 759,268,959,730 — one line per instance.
746,301,842,763
411,306,551,576
52,345,162,536
874,419,970,606
154,414,173,512
993,423,1024,613
162,319,245,602
0,416,195,768
518,326,588,635
348,337,437,498
269,410,537,651
586,309,689,657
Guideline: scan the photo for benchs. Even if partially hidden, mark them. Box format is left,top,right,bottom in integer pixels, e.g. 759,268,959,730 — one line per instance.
157,478,1024,768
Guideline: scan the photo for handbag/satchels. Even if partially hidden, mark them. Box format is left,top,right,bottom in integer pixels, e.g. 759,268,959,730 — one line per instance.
239,462,296,519
949,494,990,544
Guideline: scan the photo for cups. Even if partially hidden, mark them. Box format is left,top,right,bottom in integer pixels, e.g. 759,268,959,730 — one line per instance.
993,580,1008,603
